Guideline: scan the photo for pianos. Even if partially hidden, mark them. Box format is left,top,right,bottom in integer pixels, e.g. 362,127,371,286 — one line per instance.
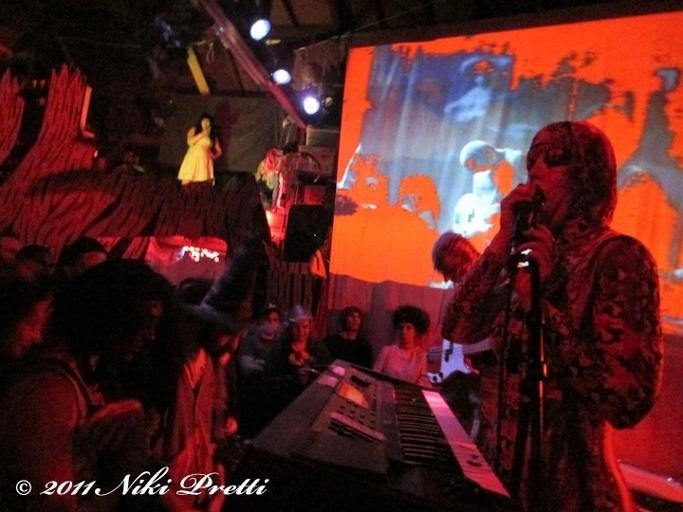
219,359,511,511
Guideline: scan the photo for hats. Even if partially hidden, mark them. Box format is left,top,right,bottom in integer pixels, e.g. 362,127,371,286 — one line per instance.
431,232,460,269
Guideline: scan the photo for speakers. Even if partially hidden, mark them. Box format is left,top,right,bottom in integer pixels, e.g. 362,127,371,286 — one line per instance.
283,204,324,263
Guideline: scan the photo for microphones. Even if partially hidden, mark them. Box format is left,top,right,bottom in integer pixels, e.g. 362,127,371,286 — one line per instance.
514,203,535,230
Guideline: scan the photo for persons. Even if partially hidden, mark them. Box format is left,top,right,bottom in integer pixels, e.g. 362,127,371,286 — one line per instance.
340,54,650,239
438,117,669,512
0,106,483,512
